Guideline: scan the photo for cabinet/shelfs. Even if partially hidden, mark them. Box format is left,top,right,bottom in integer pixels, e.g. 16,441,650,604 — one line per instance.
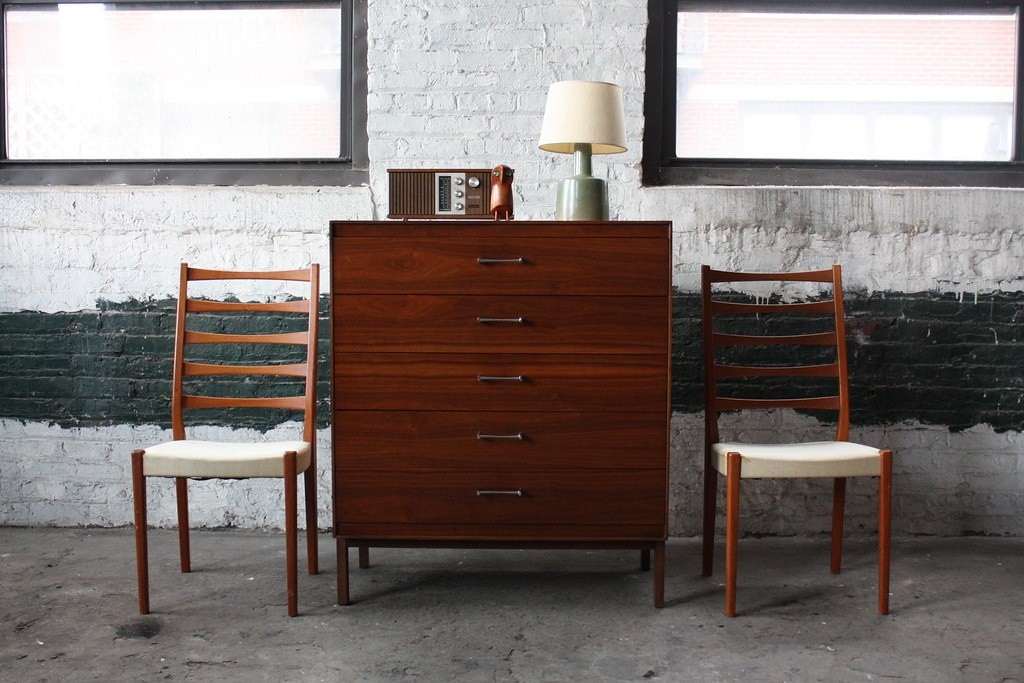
329,221,672,610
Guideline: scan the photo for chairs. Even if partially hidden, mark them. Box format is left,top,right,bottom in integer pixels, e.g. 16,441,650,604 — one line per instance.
130,262,314,618
702,264,891,612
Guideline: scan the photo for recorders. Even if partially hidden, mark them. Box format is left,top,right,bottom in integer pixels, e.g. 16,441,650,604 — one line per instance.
386,168,515,221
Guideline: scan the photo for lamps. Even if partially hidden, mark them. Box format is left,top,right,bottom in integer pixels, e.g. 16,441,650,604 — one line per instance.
535,79,627,221
